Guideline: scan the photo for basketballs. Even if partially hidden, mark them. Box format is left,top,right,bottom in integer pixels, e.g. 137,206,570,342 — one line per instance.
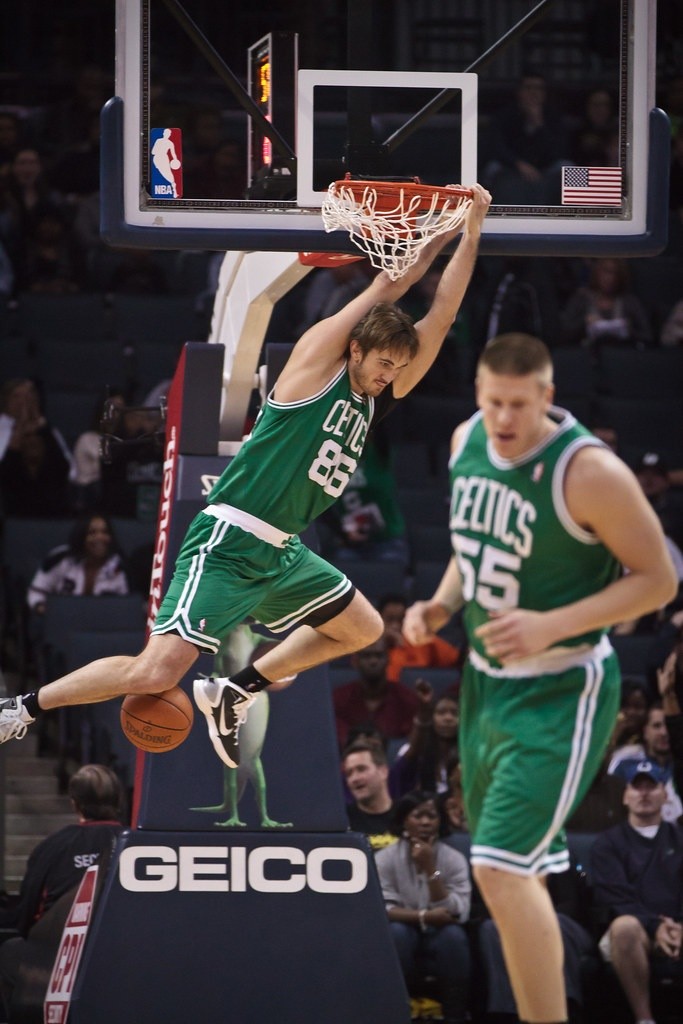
120,683,195,754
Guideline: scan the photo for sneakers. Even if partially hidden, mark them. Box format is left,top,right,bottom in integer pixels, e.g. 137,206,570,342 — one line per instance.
0,695,36,745
193,672,257,769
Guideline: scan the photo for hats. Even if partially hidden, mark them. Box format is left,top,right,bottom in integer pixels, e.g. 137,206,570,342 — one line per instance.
623,760,671,784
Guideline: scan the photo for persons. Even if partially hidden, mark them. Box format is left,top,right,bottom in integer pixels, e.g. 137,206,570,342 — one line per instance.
327,69,683,606
404,334,679,1024
0,617,680,1023
0,108,258,610
1,180,493,772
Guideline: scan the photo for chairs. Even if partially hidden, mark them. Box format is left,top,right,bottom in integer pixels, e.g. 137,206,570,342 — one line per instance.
0,254,683,986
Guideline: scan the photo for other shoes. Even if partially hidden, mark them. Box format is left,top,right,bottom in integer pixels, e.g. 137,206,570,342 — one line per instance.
441,1007,472,1024
